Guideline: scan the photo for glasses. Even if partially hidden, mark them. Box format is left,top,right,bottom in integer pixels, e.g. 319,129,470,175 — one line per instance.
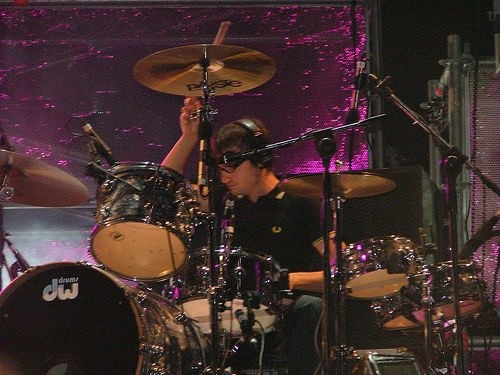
214,157,249,173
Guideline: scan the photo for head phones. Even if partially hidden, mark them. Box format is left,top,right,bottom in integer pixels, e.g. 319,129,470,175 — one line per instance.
233,119,273,169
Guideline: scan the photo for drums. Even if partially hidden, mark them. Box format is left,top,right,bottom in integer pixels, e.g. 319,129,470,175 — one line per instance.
328,346,416,375
374,260,482,331
158,244,294,338
0,261,207,375
88,162,199,282
333,234,424,300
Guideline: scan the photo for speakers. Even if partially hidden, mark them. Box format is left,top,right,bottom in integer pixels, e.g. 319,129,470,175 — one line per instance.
454,58,500,307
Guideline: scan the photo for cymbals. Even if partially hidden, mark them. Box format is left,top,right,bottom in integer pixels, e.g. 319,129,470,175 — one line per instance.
130,44,278,96
278,170,396,200
0,149,89,208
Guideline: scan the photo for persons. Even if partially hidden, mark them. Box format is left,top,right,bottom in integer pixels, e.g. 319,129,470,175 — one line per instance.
161,98,356,375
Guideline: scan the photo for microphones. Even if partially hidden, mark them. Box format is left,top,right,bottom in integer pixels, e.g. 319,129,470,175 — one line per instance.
198,120,212,188
79,118,115,165
236,309,258,349
346,57,365,125
6,241,30,271
459,210,500,260
